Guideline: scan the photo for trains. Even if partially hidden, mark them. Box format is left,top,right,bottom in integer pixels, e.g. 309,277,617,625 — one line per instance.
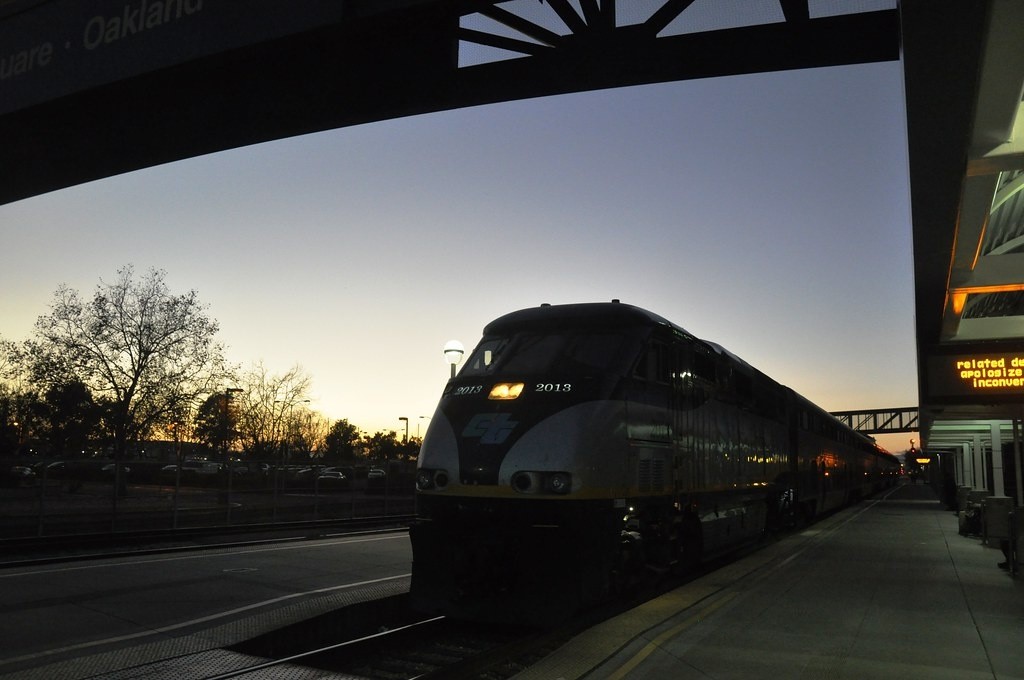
405,299,904,633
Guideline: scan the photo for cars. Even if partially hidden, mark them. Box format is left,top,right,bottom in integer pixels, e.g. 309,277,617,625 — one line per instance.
0,459,415,495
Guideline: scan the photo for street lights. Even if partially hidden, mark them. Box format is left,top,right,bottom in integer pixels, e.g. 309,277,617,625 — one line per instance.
222,388,243,485
399,417,408,459
274,399,311,470
